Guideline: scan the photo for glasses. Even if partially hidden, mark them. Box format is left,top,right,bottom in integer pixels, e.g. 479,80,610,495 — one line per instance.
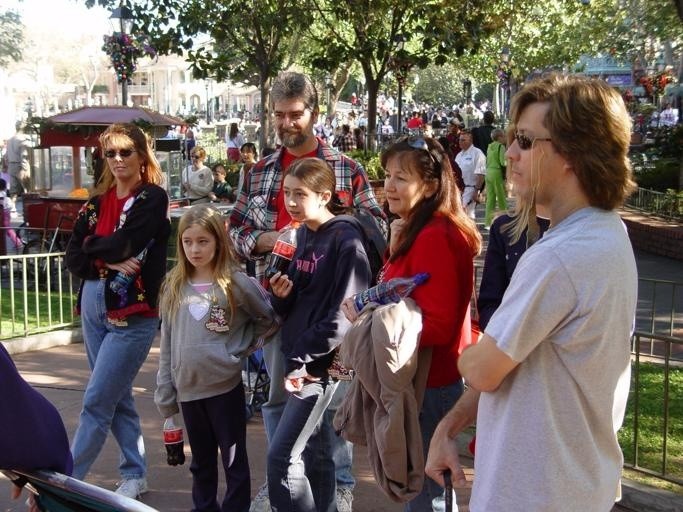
395,134,437,165
104,149,138,157
190,154,201,160
513,130,552,150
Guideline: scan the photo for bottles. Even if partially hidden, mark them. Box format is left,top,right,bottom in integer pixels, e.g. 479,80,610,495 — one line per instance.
162,414,186,466
348,273,430,314
108,237,156,297
264,221,300,279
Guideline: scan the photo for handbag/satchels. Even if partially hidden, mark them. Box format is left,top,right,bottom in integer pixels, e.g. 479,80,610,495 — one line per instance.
500,166,506,179
470,318,484,345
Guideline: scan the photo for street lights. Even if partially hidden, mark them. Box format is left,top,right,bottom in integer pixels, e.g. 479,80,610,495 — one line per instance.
324,74,332,105
394,35,405,131
103,7,143,107
463,73,471,105
203,79,211,125
646,66,658,105
414,74,421,106
496,48,514,124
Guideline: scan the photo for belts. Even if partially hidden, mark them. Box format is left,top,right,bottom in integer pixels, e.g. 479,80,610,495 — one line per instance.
465,185,475,187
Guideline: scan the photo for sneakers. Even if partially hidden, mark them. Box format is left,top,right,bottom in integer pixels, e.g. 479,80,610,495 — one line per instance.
250,483,272,511
114,477,148,499
336,488,352,512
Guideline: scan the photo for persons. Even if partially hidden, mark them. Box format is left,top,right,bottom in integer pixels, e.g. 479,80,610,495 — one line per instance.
421,72,641,511
0,119,33,248
166,92,511,230
618,89,680,145
224,70,392,510
0,340,76,512
154,205,284,510
477,188,559,335
342,132,484,511
60,119,172,502
265,155,375,511
